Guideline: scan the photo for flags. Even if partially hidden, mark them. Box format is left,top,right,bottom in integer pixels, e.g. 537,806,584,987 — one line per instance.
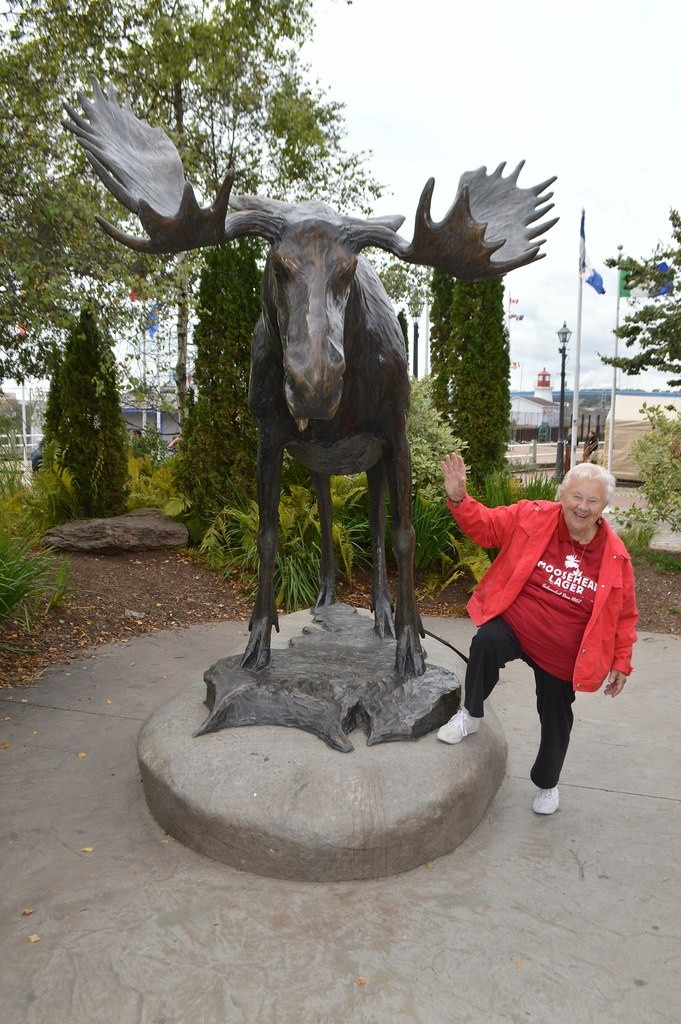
620,263,674,297
579,216,605,295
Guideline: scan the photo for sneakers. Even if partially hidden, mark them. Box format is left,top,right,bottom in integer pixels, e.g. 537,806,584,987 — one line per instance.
532,785,559,814
437,709,480,744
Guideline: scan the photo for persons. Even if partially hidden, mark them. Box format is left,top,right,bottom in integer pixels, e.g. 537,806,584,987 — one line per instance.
436,452,639,814
586,432,599,464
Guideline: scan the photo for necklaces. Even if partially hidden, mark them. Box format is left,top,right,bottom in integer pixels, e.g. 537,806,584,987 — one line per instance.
570,536,587,577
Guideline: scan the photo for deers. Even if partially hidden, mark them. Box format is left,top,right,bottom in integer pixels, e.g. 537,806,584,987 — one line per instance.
61,76,559,675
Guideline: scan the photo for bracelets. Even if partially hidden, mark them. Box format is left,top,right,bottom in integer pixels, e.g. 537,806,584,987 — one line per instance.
450,493,466,504
